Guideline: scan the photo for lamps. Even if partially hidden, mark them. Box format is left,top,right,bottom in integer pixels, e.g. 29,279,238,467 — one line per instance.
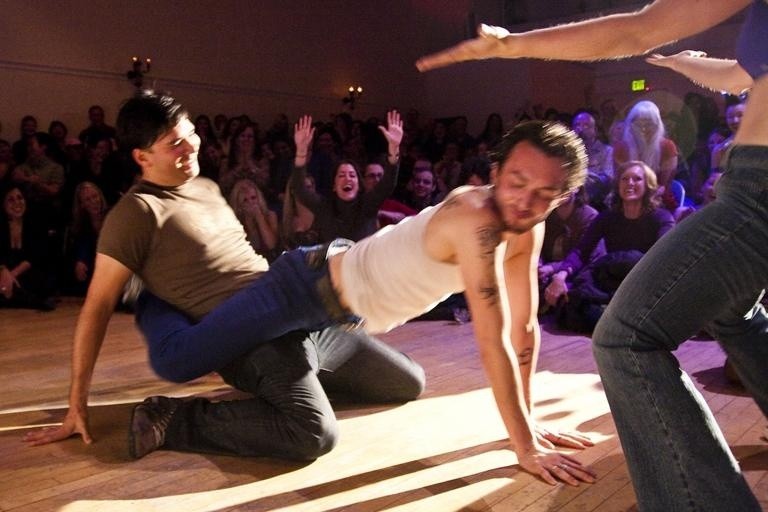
343,87,362,109
127,58,151,86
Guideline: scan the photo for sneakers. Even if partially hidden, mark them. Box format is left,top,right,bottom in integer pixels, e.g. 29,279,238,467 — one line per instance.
128,394,184,459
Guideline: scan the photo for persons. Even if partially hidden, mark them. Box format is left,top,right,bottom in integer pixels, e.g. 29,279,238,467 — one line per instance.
16,91,432,466
414,0,767,511
0,94,754,334
119,121,597,487
